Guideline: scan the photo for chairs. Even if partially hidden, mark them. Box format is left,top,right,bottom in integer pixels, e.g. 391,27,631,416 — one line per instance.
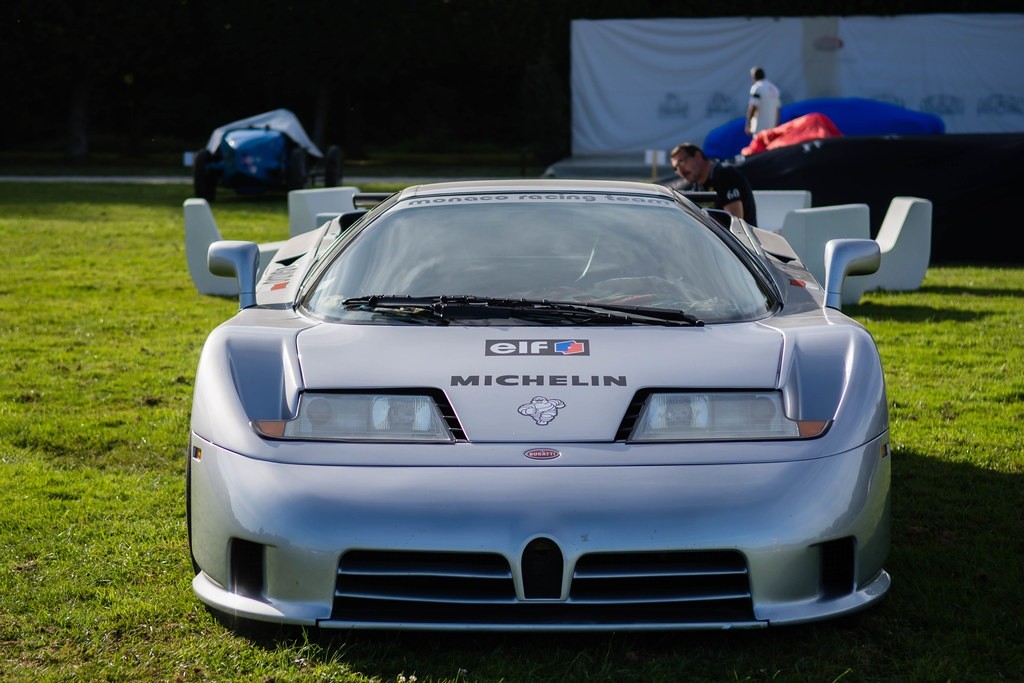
749,190,812,238
774,204,871,292
837,195,932,290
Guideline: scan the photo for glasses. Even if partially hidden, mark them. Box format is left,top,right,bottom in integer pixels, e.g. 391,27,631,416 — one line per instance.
673,154,691,173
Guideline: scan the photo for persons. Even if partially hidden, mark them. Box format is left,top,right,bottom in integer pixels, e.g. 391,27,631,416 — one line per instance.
745,66,782,139
670,143,758,228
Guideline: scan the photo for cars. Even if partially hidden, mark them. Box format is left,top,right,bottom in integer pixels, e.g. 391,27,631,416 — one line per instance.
193,122,344,205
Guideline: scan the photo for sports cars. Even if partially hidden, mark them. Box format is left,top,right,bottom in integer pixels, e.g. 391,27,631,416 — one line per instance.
178,178,937,646
